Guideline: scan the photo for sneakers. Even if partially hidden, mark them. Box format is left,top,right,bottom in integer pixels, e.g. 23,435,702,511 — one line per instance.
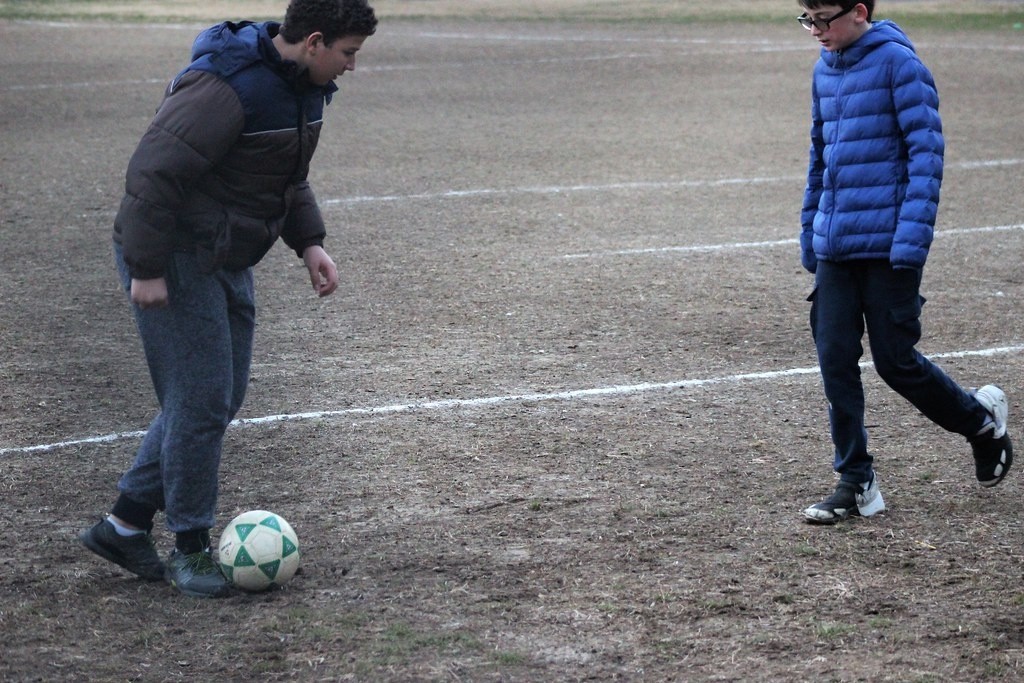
162,532,230,596
804,469,885,523
966,385,1012,487
81,517,165,582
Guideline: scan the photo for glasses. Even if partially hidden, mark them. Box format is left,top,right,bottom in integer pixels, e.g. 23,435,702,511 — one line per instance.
797,5,855,31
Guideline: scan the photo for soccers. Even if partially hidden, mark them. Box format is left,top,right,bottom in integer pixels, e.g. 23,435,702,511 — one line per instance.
217,510,300,592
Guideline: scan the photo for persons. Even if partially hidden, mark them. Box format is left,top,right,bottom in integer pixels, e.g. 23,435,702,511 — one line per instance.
81,0,379,598
797,1,1014,523
